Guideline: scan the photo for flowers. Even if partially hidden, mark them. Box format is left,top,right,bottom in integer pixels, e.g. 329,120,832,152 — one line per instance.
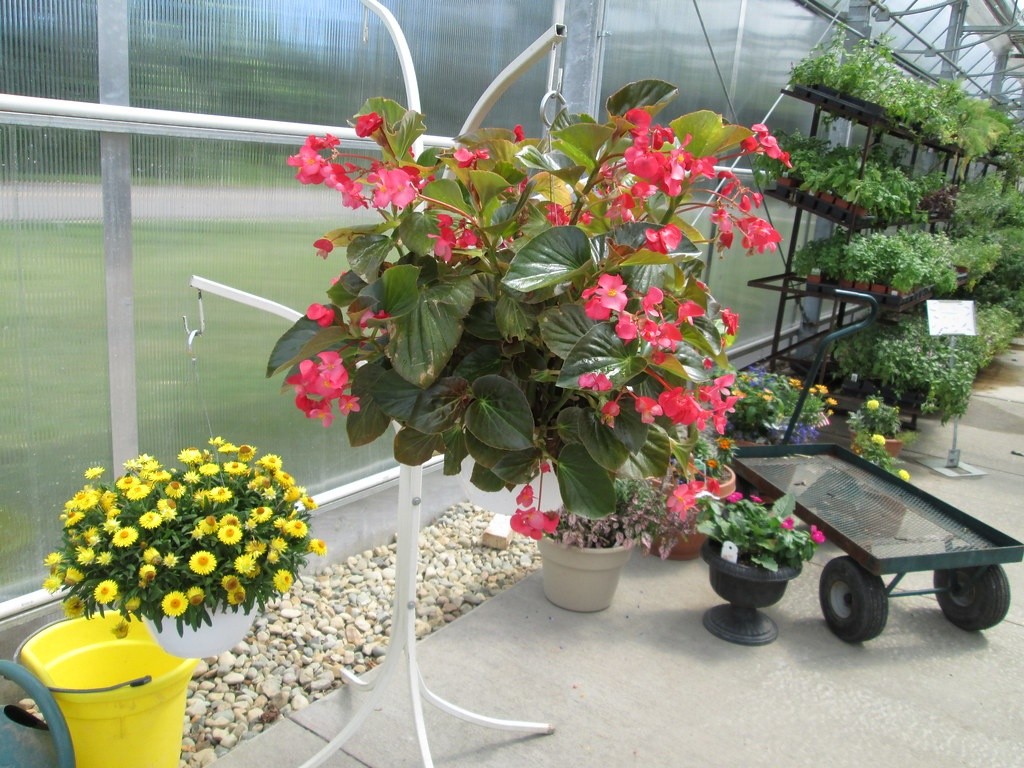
695,489,825,574
263,74,794,541
45,434,326,636
689,436,740,482
719,366,838,444
845,396,910,483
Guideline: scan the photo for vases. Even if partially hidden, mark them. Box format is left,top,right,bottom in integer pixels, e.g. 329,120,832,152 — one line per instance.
631,464,736,561
847,425,903,460
143,586,262,658
701,536,802,642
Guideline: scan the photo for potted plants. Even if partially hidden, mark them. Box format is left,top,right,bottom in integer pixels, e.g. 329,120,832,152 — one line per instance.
538,477,700,612
753,19,1024,426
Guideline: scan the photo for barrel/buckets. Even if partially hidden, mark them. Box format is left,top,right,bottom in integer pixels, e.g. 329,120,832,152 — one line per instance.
11,608,199,768
